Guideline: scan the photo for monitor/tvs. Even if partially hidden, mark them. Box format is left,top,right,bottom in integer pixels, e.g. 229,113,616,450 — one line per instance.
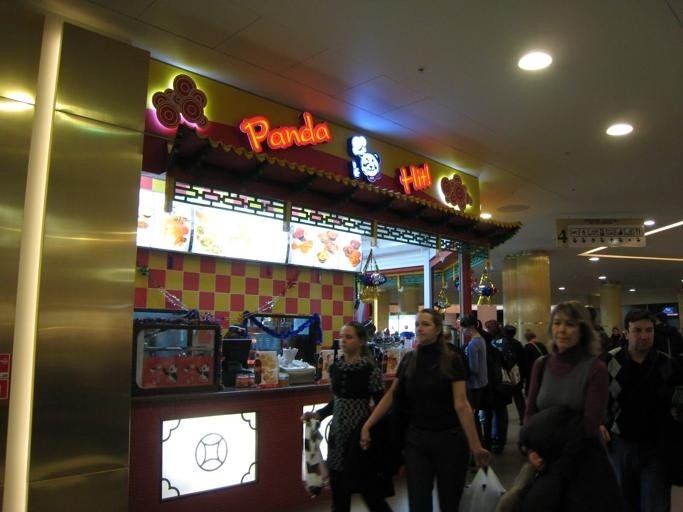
222,338,252,386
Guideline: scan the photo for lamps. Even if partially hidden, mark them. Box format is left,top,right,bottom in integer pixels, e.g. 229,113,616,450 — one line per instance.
474,266,497,297
359,248,386,287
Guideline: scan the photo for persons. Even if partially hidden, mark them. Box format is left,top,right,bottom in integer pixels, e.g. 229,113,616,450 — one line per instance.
299,301,683,512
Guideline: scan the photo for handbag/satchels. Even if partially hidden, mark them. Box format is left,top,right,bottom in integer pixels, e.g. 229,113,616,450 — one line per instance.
380,383,410,431
499,365,521,385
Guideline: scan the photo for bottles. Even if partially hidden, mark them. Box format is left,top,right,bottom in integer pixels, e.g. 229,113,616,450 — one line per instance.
317,352,323,379
254,353,262,384
383,350,387,373
